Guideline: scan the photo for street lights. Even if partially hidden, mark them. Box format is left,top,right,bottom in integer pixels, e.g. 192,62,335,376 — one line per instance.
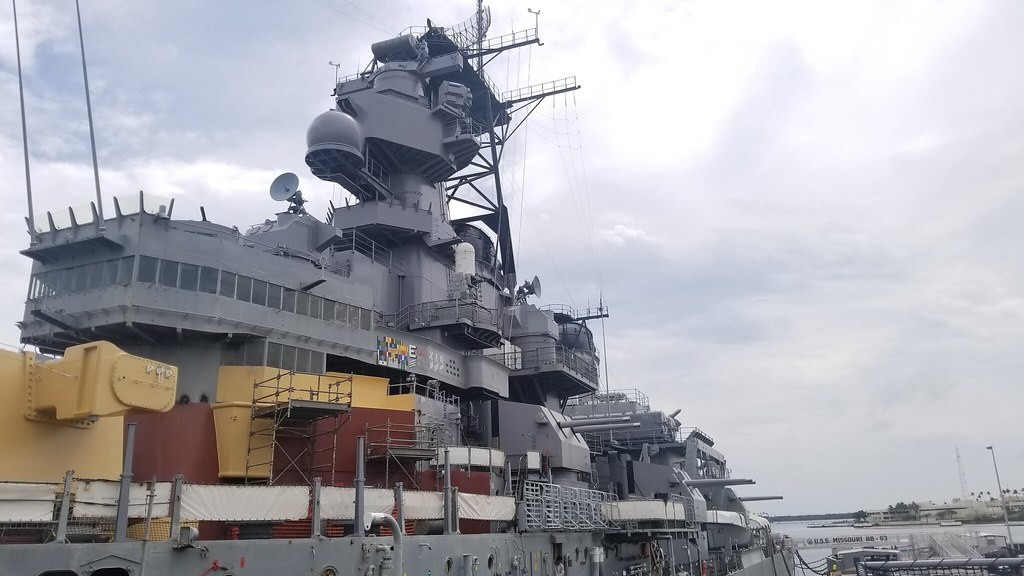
987,445,1015,550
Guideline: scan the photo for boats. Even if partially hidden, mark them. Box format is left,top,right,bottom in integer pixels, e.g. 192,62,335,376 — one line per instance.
0,0,784,576
